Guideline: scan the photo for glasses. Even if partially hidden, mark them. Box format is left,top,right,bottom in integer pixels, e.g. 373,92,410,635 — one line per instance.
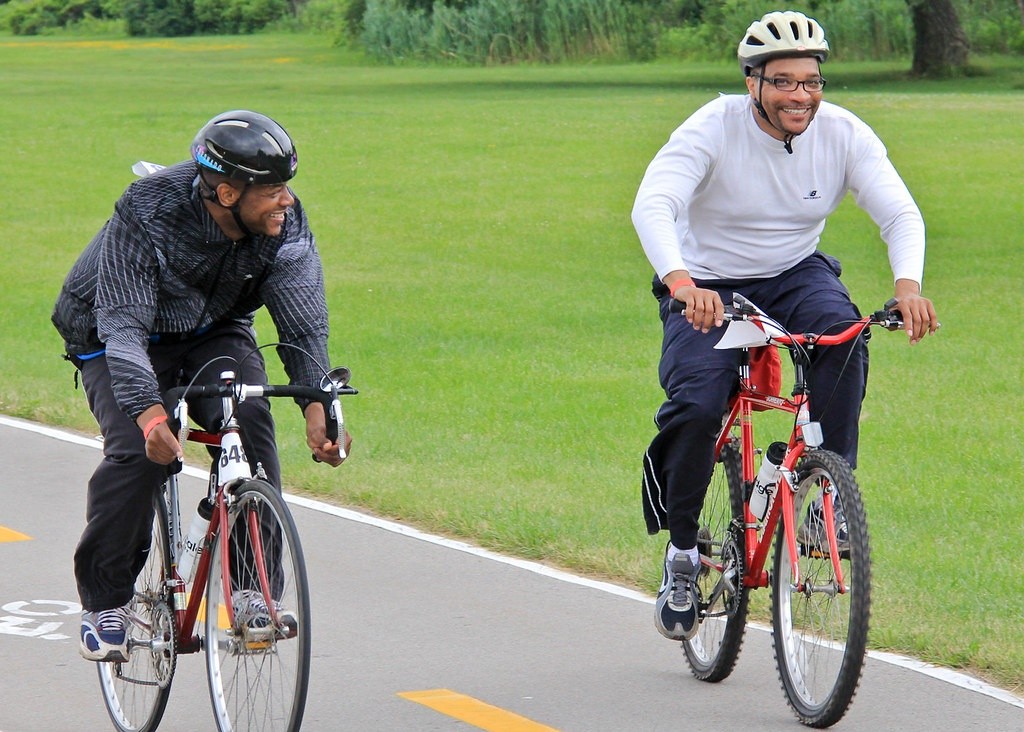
750,75,827,92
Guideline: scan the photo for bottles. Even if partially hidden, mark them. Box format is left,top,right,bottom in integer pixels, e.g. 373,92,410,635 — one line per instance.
749,442,789,519
178,497,215,581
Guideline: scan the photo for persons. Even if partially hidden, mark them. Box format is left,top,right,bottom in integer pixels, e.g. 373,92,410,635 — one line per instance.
630,11,937,641
51,110,350,661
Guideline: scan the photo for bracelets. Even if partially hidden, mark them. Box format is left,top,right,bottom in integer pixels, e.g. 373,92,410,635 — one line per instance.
671,281,697,296
143,415,168,439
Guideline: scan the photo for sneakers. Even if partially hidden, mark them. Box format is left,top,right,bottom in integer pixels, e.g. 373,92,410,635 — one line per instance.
229,591,297,641
654,538,702,640
79,603,131,663
795,499,850,558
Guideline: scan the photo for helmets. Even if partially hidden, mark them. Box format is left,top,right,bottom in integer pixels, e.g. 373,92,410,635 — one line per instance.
191,109,297,186
738,10,831,75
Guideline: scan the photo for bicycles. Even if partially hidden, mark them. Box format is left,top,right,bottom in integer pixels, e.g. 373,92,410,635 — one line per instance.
95,343,359,732
668,295,940,727
